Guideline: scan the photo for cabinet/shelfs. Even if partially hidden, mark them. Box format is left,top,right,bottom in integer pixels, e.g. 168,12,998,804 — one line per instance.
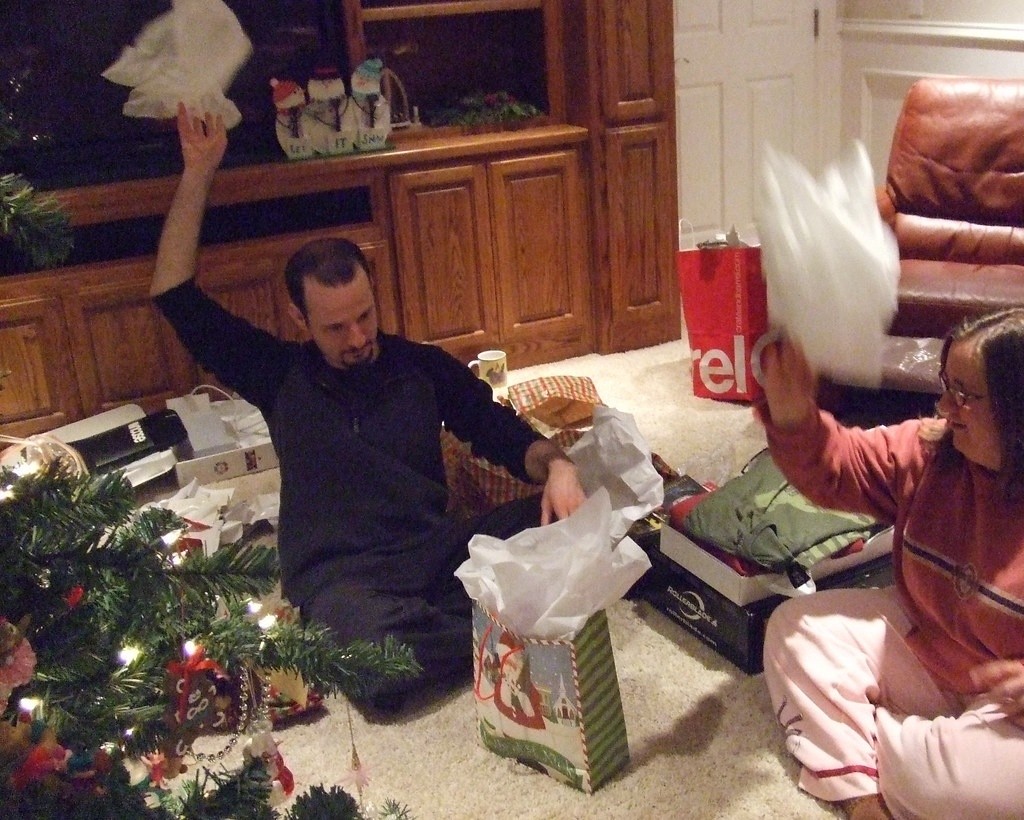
0,123,596,451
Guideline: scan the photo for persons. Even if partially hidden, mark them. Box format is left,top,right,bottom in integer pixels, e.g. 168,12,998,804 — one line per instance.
762,303,1024,820
150,102,590,721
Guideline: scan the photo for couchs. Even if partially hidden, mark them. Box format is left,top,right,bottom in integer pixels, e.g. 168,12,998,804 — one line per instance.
816,76,1024,430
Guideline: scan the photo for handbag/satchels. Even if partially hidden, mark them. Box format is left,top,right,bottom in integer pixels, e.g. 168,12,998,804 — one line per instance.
677,215,782,405
506,371,609,432
469,595,633,795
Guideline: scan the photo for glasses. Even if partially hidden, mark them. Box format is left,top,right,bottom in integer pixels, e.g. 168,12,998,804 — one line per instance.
936,367,992,408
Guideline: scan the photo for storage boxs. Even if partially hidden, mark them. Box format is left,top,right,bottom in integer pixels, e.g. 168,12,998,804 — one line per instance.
167,399,280,489
644,486,894,677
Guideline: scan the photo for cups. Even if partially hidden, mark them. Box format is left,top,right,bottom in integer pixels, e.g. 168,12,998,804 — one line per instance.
468,349,508,388
